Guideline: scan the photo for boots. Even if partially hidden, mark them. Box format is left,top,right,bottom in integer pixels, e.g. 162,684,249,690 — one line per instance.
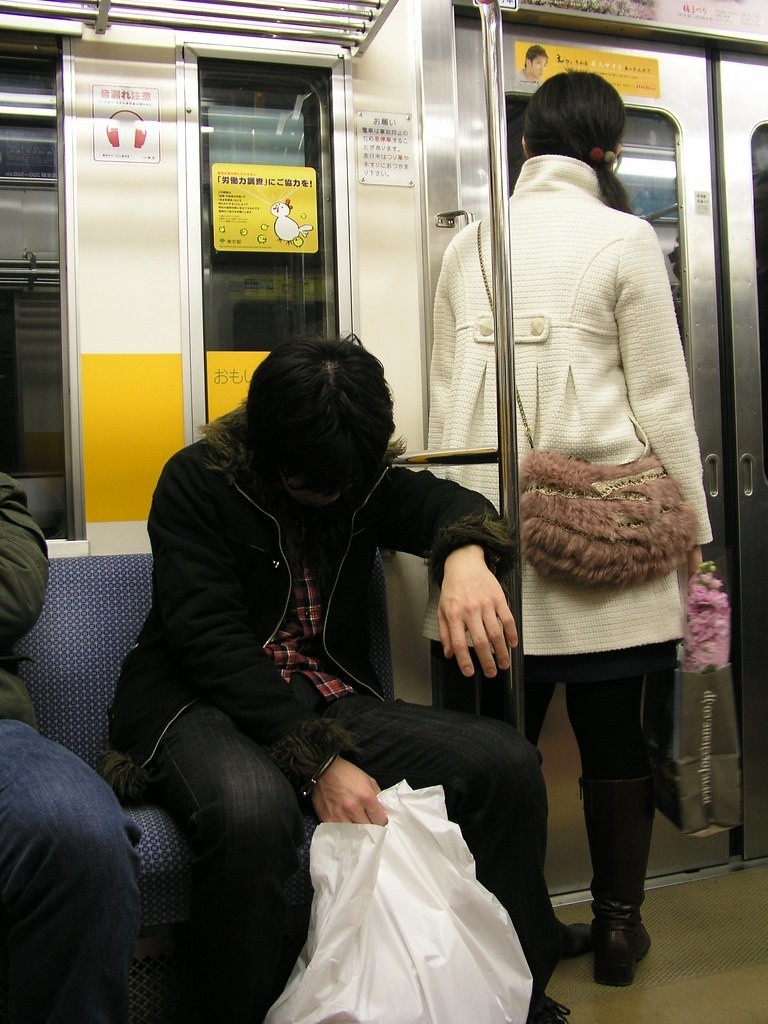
559,922,591,959
577,765,656,987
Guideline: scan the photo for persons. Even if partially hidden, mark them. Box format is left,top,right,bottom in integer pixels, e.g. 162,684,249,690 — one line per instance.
516,45,549,92
425,70,715,987
100,333,578,1023
0,469,141,1024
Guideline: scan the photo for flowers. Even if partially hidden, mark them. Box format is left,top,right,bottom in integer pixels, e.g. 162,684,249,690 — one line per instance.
678,560,732,676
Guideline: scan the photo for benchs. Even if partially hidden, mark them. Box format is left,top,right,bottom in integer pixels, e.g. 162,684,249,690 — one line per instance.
13,553,193,933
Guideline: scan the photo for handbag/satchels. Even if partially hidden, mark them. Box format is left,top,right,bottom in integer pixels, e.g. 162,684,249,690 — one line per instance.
264,779,533,1023
520,444,697,586
